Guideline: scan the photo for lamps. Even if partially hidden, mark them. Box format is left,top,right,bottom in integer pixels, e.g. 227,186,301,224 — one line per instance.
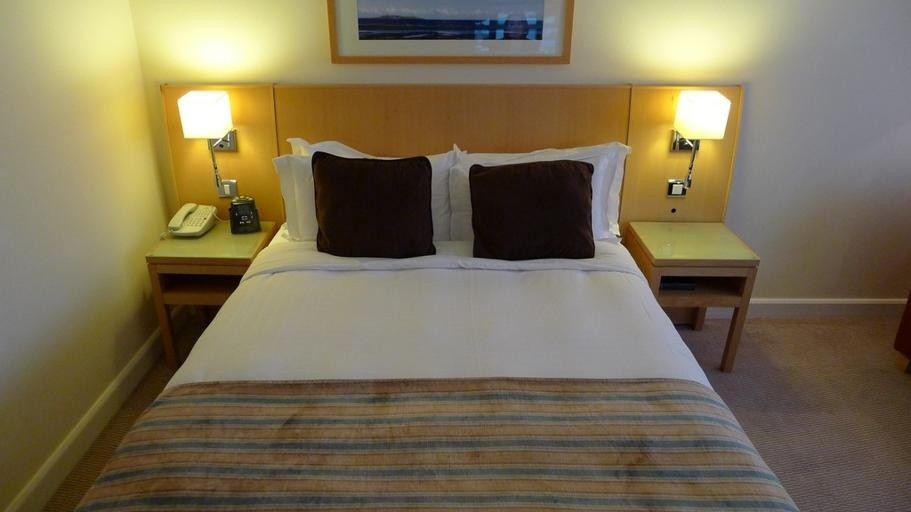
665,91,732,197
177,88,239,188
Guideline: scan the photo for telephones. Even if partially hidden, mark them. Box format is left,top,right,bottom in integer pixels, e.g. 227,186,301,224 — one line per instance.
168,202,218,237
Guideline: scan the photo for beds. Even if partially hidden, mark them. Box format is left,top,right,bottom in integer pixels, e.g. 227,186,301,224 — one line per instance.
76,215,804,511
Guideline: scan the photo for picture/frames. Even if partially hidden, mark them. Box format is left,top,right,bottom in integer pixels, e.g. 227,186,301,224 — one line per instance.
326,0,577,67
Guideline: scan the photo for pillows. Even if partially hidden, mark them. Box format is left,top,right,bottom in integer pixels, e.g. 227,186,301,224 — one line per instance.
453,142,632,242
273,138,453,241
469,159,595,259
311,150,437,259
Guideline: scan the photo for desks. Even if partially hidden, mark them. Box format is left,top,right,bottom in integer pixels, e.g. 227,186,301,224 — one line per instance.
145,219,278,363
627,220,763,375
